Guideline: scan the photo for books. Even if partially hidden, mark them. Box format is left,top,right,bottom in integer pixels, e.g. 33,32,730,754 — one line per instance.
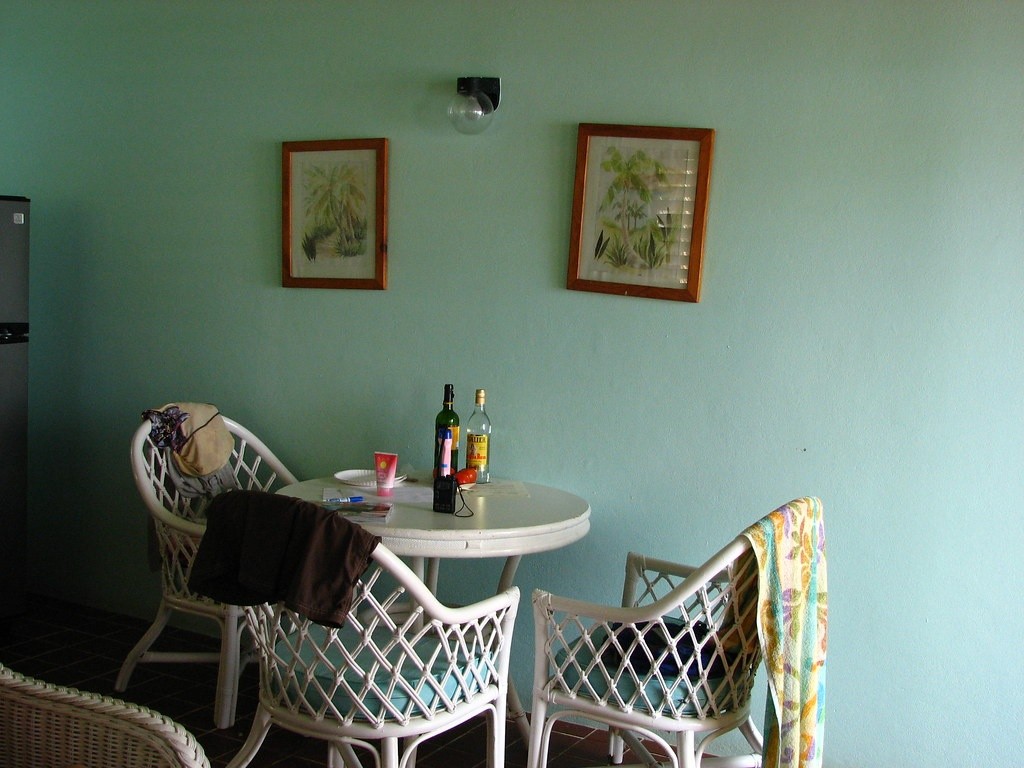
312,501,393,524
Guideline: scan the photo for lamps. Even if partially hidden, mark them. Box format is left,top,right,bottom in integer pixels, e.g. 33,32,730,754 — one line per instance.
447,76,502,136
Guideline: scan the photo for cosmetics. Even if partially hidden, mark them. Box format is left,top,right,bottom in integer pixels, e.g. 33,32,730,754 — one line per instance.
374,451,399,498
438,428,453,477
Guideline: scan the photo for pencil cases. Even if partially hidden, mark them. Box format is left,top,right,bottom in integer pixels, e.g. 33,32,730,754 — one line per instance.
319,487,395,523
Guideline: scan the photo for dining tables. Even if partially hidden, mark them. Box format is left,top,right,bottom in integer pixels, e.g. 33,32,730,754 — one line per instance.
274,470,591,768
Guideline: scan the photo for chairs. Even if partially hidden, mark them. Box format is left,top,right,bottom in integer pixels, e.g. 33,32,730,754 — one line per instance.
115,402,300,729
207,490,522,768
525,496,824,768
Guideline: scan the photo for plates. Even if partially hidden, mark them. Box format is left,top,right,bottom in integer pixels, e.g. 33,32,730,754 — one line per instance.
334,469,407,487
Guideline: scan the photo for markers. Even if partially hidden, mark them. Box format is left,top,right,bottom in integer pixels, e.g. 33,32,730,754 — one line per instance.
327,497,364,503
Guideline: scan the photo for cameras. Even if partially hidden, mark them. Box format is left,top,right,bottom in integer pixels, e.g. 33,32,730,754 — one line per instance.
433,475,458,513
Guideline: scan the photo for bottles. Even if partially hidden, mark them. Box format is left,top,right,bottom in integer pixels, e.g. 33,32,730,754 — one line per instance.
466,389,491,484
433,384,460,477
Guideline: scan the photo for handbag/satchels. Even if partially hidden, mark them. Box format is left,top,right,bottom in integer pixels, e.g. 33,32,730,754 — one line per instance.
602,621,712,677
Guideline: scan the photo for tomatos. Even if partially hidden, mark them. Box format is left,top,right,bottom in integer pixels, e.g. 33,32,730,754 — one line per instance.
456,468,477,484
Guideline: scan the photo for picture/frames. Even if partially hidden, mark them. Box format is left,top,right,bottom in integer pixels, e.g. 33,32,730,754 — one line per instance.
282,137,389,291
565,122,716,303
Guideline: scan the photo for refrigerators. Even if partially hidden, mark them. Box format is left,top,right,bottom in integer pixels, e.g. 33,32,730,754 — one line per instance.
0,195,31,613
